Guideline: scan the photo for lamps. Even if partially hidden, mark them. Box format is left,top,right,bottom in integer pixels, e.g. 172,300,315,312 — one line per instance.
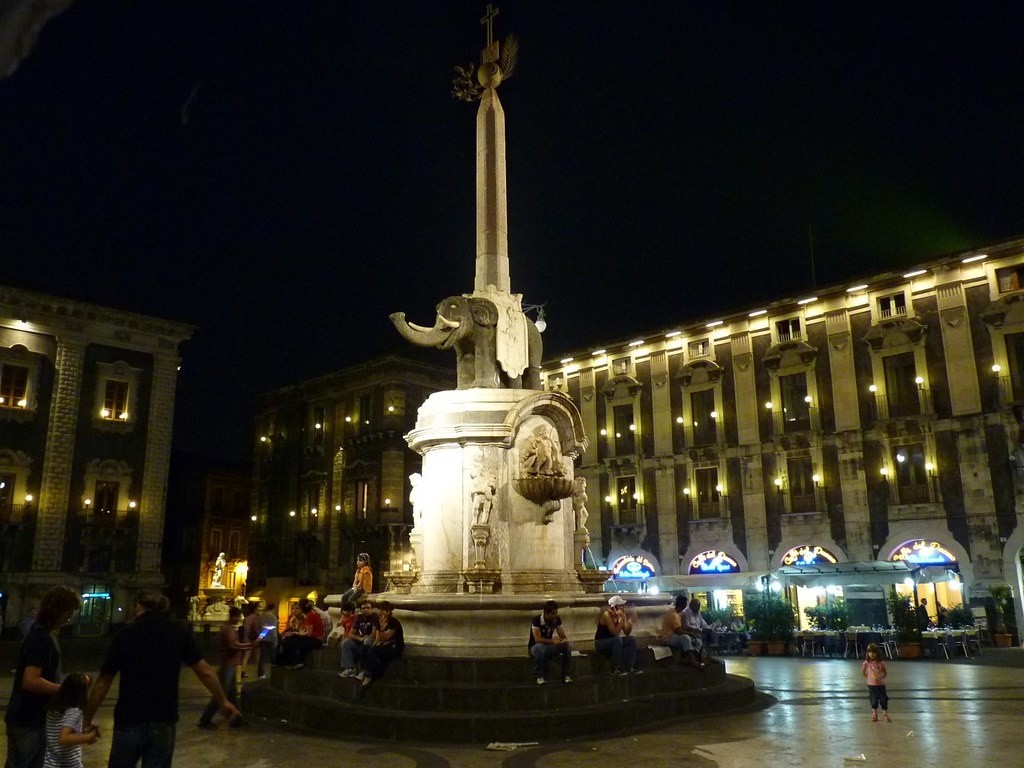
868,385,878,398
600,424,645,507
1009,456,1018,471
804,396,813,409
0,397,137,513
677,417,684,429
711,411,718,424
915,377,925,391
766,401,774,416
812,474,821,488
926,463,934,477
683,488,692,502
775,479,786,496
522,301,547,333
250,405,395,526
992,364,1003,381
716,485,729,497
879,468,889,485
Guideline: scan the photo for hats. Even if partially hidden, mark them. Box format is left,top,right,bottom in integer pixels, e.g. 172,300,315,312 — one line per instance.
608,595,627,608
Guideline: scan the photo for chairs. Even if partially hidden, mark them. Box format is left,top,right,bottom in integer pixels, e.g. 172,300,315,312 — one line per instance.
718,629,983,661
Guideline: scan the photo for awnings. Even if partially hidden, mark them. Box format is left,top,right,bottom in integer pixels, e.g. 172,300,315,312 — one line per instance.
780,560,912,577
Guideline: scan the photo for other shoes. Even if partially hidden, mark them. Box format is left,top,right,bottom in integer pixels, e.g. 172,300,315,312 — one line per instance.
338,669,372,690
627,667,644,675
872,713,878,721
562,676,573,684
883,715,891,722
198,721,217,730
536,677,546,685
611,670,627,677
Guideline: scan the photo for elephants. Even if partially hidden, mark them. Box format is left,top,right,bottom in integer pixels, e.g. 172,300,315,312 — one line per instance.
389,296,543,391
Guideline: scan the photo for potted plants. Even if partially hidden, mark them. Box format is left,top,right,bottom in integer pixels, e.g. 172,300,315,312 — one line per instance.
992,586,1012,648
871,588,923,660
765,596,796,657
746,603,766,656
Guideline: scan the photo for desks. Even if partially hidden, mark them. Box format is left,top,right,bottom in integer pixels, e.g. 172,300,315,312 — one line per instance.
794,629,976,657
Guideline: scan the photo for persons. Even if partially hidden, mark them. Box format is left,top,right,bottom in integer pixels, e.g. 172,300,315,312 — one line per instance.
337,553,374,626
470,460,497,525
17,608,38,653
43,672,98,768
4,584,82,768
574,476,589,530
197,595,405,728
527,600,575,686
920,598,929,630
534,425,554,476
213,552,227,583
83,591,246,768
409,473,422,532
594,595,644,676
662,595,720,670
861,643,891,722
937,601,947,626
